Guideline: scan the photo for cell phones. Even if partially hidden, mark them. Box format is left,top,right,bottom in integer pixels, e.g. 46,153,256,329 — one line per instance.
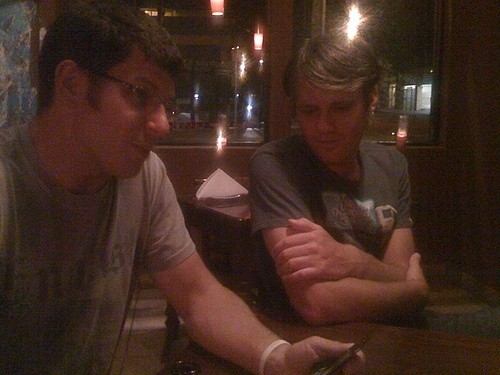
312,335,371,375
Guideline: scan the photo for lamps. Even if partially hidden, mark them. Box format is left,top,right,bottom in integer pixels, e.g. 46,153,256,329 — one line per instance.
253,23,264,51
395,115,408,148
210,0,224,16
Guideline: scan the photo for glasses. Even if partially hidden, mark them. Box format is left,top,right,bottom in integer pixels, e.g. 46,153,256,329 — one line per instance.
79,63,179,123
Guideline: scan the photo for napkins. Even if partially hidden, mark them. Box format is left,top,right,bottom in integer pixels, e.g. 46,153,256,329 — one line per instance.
195,168,249,199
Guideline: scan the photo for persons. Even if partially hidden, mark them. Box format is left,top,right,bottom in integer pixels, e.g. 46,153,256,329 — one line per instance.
0,0,366,375
248,33,430,325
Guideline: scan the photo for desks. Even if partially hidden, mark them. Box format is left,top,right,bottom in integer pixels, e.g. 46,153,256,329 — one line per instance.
160,177,252,365
155,302,500,375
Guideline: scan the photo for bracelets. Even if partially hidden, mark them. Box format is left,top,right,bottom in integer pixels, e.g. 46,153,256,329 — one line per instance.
258,340,291,375
